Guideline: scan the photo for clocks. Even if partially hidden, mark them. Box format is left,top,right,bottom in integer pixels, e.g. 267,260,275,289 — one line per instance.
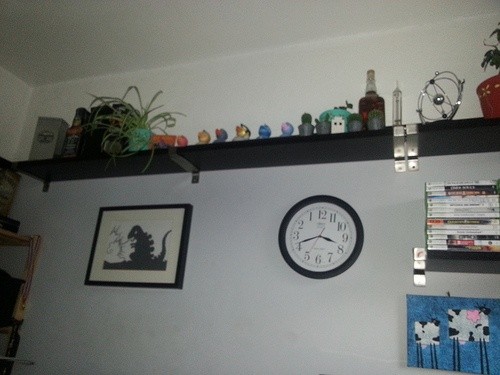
277,195,365,279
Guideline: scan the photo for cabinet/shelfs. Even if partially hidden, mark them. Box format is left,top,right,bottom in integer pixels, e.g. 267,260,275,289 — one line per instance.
0,228,43,375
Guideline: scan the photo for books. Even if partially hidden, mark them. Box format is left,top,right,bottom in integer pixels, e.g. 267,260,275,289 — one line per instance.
425,180,500,252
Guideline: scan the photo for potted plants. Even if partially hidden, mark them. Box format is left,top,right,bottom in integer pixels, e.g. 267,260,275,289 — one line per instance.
298,113,313,136
78,85,187,176
367,110,384,130
314,115,331,134
476,20,500,119
347,112,362,133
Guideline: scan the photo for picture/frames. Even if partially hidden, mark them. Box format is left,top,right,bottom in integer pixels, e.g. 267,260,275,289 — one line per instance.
84,204,193,290
